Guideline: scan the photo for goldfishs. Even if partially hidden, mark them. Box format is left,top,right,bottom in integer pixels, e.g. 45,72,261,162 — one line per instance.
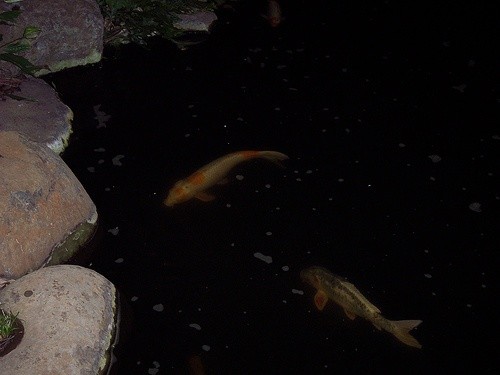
163,150,289,207
301,266,423,349
